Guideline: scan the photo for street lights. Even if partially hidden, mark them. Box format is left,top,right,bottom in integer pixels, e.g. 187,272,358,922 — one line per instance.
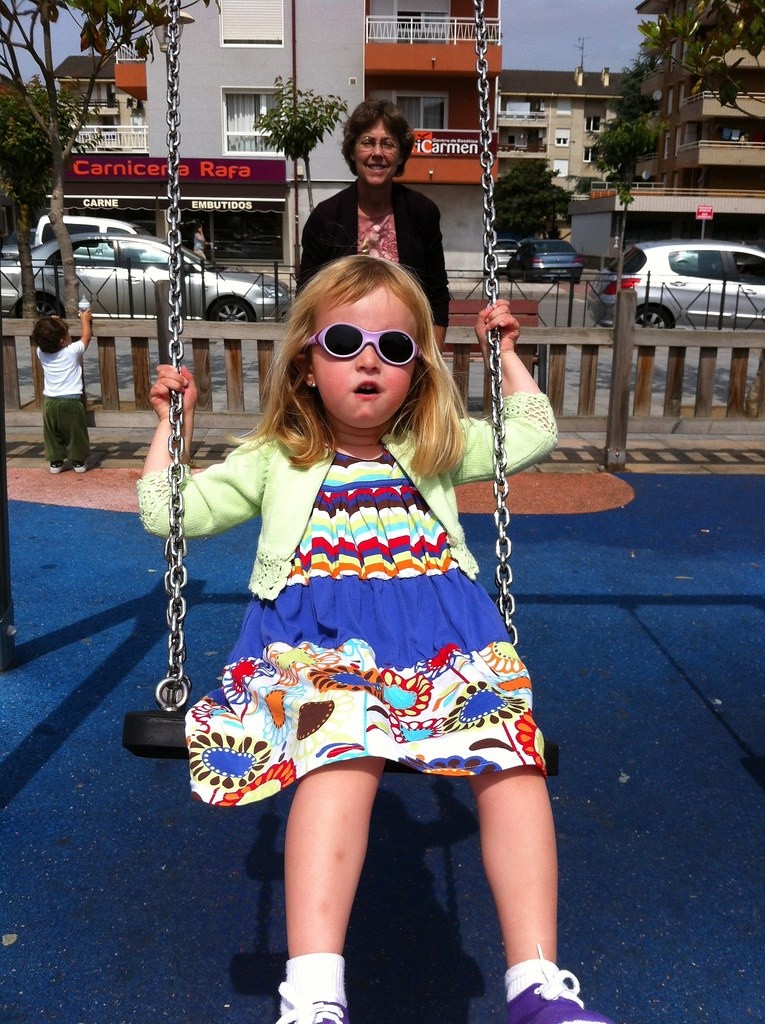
151,9,196,84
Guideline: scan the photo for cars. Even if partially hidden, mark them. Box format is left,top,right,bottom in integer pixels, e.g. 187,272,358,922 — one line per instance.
491,240,521,272
586,238,764,330
506,240,583,285
1,231,290,322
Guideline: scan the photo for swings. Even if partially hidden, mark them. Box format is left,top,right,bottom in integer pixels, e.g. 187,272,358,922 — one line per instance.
119,0,564,779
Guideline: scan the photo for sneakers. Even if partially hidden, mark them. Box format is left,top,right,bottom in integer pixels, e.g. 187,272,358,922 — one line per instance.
506,943,614,1024
277,981,349,1024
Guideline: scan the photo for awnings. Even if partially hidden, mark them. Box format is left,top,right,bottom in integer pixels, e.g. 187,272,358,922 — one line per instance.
45,184,287,213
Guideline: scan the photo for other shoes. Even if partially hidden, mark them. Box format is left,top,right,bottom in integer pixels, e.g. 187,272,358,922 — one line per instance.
49,460,67,473
72,461,87,472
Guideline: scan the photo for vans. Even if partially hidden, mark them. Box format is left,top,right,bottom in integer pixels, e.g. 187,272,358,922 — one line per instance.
35,215,167,262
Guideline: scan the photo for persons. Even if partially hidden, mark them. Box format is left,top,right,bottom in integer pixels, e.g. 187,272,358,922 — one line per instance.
194,225,206,259
30,309,93,474
295,101,450,359
137,256,615,1024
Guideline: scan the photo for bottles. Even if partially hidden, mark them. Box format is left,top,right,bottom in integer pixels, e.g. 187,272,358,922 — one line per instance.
79,295,90,317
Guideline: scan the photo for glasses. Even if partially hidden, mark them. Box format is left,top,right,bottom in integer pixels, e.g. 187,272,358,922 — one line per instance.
302,322,423,365
355,137,400,153
